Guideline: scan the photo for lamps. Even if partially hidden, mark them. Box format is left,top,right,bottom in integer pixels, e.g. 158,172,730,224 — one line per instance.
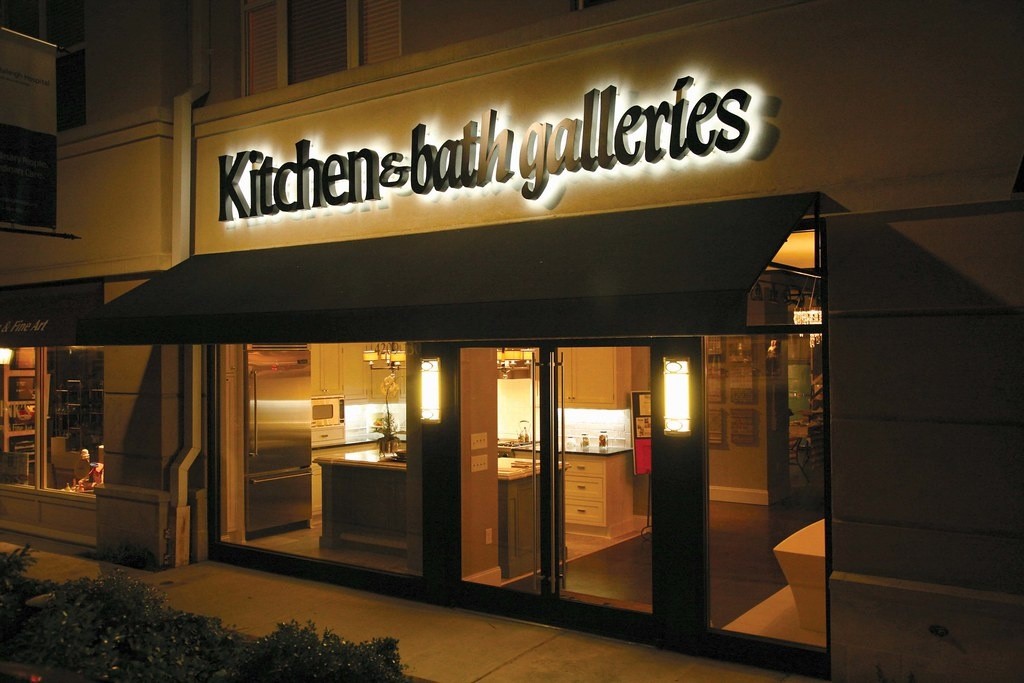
662,356,691,438
0,346,13,366
497,348,532,379
363,342,405,378
419,356,441,424
794,277,822,349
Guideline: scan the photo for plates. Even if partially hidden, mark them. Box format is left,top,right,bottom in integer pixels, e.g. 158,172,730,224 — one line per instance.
398,459,407,462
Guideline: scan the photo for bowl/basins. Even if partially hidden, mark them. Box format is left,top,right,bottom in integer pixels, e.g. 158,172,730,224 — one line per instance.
395,450,407,458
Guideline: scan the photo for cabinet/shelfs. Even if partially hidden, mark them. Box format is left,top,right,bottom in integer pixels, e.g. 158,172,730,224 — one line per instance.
310,342,633,578
4,347,104,464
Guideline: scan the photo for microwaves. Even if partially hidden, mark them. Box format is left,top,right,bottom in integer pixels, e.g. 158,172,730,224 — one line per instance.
311,394,346,428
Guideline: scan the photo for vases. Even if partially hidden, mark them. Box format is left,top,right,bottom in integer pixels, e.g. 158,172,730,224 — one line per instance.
380,441,398,454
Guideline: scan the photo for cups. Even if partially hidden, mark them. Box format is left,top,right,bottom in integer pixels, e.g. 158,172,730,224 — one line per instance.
98,445,104,464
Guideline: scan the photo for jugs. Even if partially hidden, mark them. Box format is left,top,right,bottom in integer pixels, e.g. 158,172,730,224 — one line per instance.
516,420,531,445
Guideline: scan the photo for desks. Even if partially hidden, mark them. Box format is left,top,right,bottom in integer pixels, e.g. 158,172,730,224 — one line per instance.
789,421,810,484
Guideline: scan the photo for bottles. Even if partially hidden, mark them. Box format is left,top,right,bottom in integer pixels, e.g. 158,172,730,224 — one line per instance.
567,436,575,450
766,339,779,375
580,433,590,448
79,449,90,481
598,430,608,450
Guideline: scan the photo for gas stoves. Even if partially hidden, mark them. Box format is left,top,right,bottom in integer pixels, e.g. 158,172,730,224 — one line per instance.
497,439,540,448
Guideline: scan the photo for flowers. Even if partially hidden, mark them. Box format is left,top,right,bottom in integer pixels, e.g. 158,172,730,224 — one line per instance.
369,375,407,442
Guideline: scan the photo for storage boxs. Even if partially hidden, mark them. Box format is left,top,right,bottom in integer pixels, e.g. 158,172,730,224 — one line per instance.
15,379,33,399
11,348,35,369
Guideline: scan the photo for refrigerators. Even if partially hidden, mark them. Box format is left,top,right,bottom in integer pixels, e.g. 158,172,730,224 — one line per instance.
243,343,313,540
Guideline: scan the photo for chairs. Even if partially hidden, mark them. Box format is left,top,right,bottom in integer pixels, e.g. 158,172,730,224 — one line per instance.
51,437,90,488
